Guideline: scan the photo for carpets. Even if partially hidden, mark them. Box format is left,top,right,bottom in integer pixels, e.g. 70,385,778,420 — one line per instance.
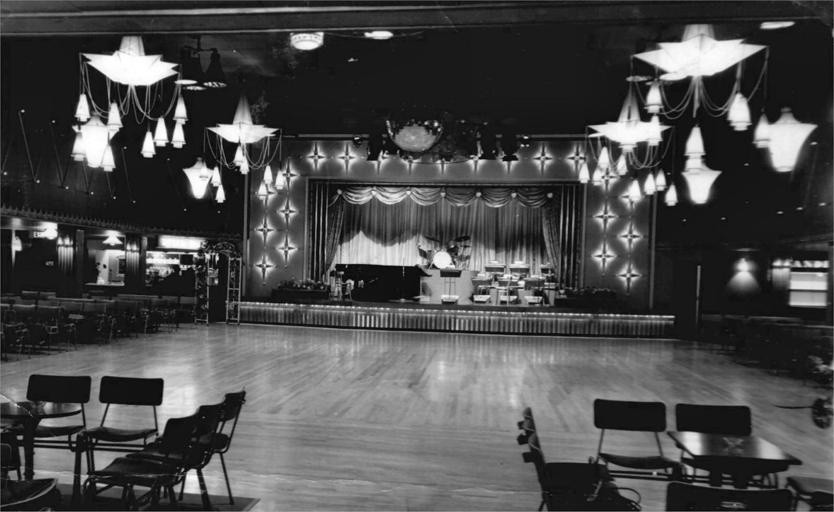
45,483,261,510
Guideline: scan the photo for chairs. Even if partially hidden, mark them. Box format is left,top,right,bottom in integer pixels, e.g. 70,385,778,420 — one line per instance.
674,400,755,489
589,399,682,484
0,374,246,511
516,401,641,510
663,480,798,510
785,473,832,511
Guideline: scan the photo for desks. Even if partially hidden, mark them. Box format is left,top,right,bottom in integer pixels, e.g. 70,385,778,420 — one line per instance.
666,430,804,488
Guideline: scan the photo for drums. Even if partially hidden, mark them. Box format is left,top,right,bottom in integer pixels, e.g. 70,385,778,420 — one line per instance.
428,251,452,269
447,245,459,256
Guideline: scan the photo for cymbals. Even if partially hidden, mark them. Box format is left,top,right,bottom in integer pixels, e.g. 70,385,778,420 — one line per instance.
460,245,471,247
424,235,440,242
455,235,470,241
